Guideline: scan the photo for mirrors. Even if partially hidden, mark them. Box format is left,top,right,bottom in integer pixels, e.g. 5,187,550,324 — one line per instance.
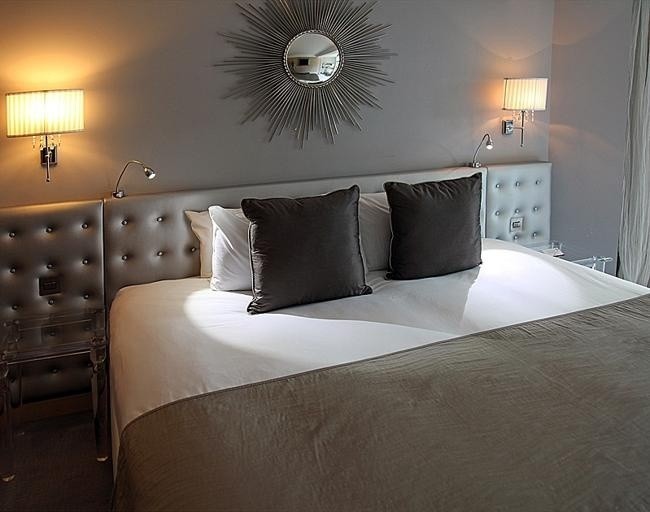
212,0,399,148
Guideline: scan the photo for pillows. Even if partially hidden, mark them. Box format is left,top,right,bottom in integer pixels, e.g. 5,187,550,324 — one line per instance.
184,210,213,277
241,184,373,314
383,172,483,279
358,192,391,271
209,206,252,290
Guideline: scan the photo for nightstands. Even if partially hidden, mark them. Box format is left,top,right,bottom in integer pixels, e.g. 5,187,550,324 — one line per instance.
525,240,613,273
0,309,110,482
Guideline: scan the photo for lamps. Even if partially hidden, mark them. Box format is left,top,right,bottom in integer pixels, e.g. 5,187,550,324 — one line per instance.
5,89,86,182
472,134,494,167
501,78,549,147
112,160,156,199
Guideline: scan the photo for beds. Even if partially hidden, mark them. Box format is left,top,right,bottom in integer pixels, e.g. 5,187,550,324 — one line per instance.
103,167,650,512
